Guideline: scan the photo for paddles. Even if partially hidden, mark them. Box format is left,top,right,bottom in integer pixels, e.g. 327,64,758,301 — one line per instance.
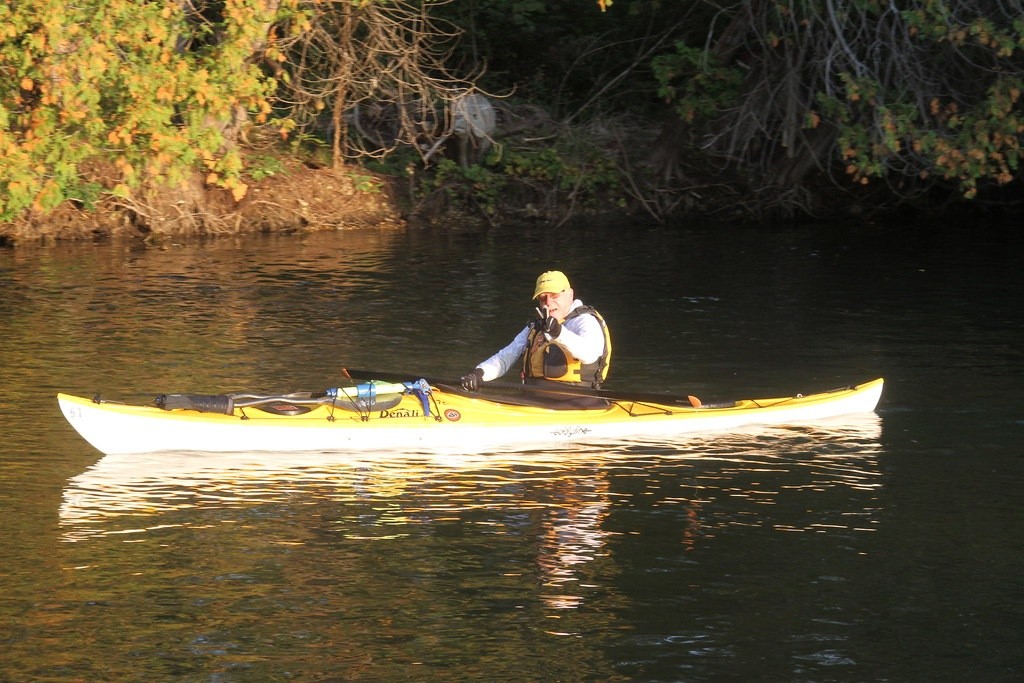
339,365,737,409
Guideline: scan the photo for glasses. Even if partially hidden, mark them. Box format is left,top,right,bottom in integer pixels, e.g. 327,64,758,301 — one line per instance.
535,292,562,301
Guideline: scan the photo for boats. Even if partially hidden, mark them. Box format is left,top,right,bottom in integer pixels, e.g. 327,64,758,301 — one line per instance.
55,369,886,458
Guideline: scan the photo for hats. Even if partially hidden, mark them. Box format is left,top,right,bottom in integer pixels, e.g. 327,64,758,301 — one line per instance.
532,271,571,301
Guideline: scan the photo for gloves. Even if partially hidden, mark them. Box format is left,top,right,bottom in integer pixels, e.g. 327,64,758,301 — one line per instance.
535,306,562,339
459,368,486,392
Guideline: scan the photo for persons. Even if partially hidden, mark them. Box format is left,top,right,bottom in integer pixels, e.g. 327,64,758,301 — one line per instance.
460,270,612,392
